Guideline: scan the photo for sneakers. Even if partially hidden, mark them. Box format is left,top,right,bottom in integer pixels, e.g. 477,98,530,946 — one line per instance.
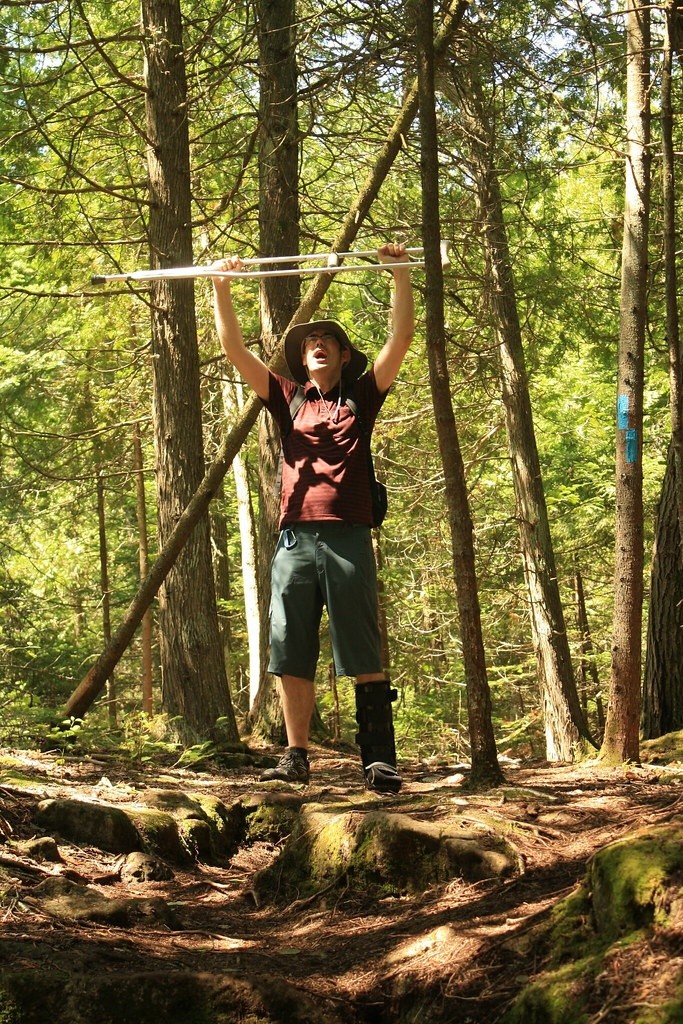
259,746,309,785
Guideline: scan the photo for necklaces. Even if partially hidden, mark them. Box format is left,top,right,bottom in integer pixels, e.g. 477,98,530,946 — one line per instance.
309,380,342,421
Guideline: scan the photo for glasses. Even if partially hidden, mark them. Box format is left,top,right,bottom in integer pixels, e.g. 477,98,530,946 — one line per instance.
304,333,334,347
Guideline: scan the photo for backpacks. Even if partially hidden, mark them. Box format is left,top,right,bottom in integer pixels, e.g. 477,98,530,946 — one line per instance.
280,387,388,528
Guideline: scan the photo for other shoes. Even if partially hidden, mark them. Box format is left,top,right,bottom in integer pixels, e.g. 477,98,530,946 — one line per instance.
363,756,403,788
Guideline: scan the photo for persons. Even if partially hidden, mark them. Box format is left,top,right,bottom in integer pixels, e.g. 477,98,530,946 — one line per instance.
210,242,420,790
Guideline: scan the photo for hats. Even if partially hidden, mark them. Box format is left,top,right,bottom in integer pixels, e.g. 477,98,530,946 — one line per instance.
284,320,367,387
